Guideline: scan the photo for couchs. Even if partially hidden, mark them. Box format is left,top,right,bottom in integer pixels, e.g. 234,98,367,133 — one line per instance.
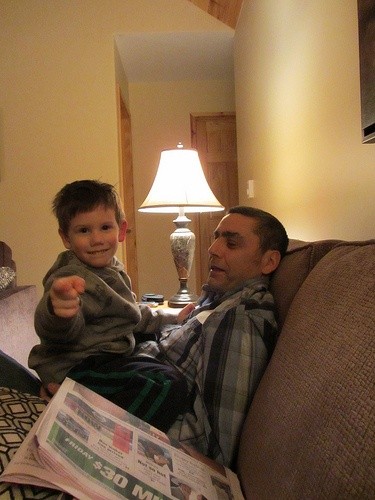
0,239,375,500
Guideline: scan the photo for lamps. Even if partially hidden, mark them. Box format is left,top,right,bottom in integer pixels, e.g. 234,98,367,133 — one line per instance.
137,141,227,308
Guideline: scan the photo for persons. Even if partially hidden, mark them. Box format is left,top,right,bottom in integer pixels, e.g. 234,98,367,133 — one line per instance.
27,178,195,433
171,478,194,500
1,205,290,466
